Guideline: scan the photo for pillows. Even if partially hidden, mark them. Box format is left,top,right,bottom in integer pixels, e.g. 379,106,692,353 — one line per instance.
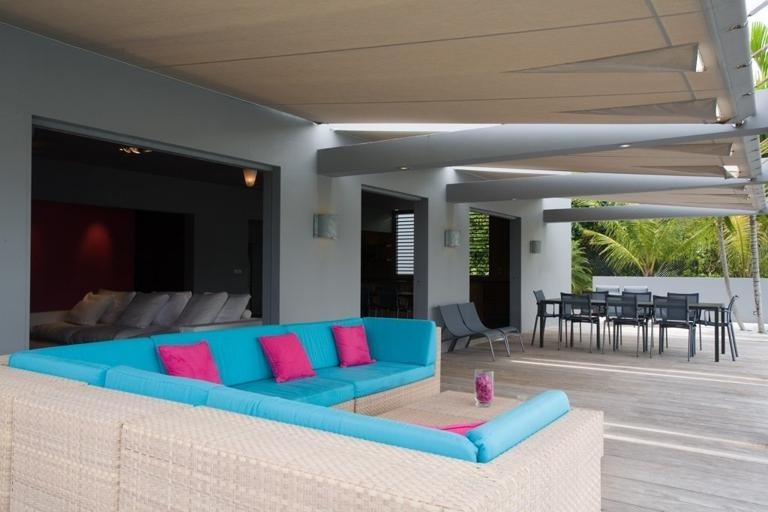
330,324,377,367
69,289,250,330
158,340,222,385
256,332,317,384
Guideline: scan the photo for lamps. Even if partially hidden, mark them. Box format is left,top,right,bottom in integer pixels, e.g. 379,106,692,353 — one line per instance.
242,169,258,187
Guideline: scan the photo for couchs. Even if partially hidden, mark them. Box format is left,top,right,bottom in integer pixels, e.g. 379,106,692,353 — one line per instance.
0,315,603,511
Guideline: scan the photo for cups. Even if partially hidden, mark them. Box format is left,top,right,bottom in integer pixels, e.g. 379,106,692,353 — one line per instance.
474,368,495,407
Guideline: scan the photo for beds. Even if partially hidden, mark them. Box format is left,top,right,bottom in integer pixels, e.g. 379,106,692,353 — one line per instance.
30,308,263,345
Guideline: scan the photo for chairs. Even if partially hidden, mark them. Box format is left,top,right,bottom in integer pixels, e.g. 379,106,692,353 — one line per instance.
531,286,741,362
451,302,525,357
437,304,510,361
